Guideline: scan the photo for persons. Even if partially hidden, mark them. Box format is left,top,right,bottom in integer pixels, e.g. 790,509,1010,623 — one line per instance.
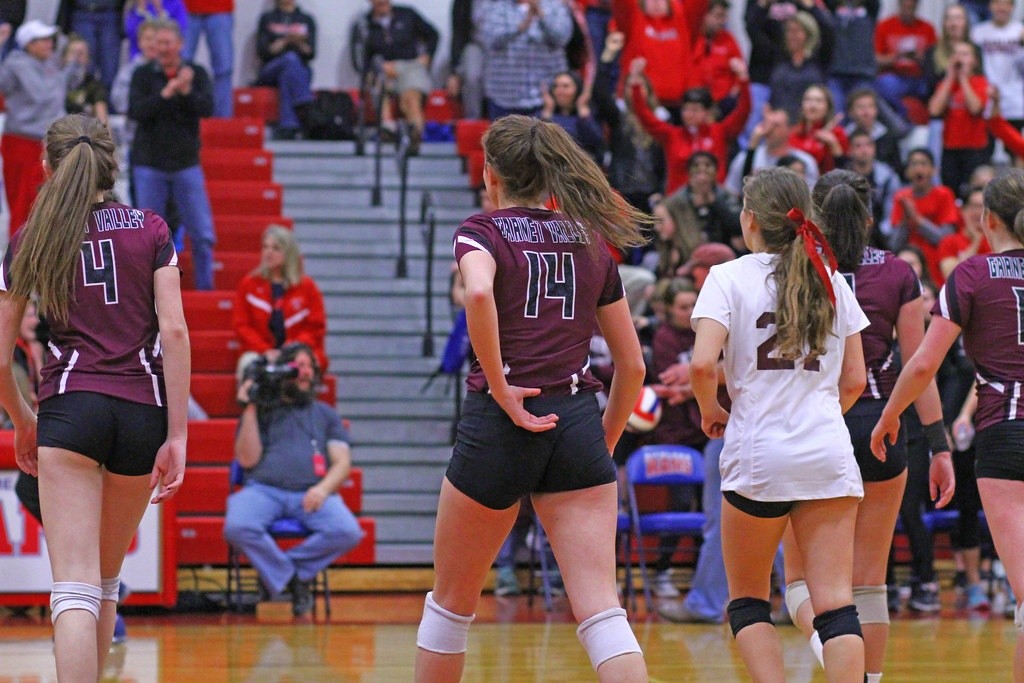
0,0,1024,683
413,114,667,683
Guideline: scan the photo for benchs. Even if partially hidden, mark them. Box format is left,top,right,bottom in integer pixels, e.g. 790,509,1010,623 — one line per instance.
0,85,629,596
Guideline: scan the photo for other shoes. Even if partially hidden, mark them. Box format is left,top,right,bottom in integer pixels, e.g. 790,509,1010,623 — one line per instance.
295,104,333,130
657,598,721,623
379,121,397,142
287,577,314,617
884,568,1023,618
536,572,567,596
241,588,266,615
493,566,521,597
404,123,425,144
768,606,798,626
277,127,313,140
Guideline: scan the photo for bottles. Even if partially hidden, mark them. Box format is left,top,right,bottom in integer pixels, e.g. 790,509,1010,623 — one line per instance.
957,426,971,452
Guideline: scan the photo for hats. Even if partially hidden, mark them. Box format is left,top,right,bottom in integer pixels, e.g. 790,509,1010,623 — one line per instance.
15,20,58,50
677,243,737,276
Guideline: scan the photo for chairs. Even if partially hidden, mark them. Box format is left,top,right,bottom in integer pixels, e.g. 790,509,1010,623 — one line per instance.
527,444,985,614
226,455,335,623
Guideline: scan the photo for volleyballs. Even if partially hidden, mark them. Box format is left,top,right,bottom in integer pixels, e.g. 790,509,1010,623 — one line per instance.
625,384,662,433
